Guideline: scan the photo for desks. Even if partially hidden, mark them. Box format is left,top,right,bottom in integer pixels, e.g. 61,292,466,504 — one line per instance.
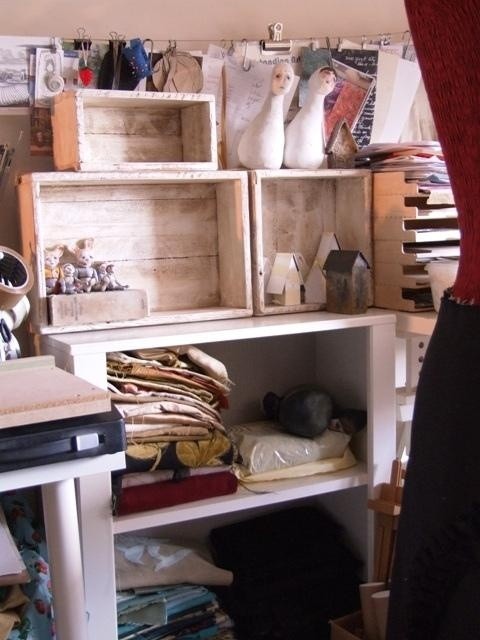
0,366,128,639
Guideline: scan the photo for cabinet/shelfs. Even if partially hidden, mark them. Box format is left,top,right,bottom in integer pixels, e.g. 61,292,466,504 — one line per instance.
40,308,398,640
